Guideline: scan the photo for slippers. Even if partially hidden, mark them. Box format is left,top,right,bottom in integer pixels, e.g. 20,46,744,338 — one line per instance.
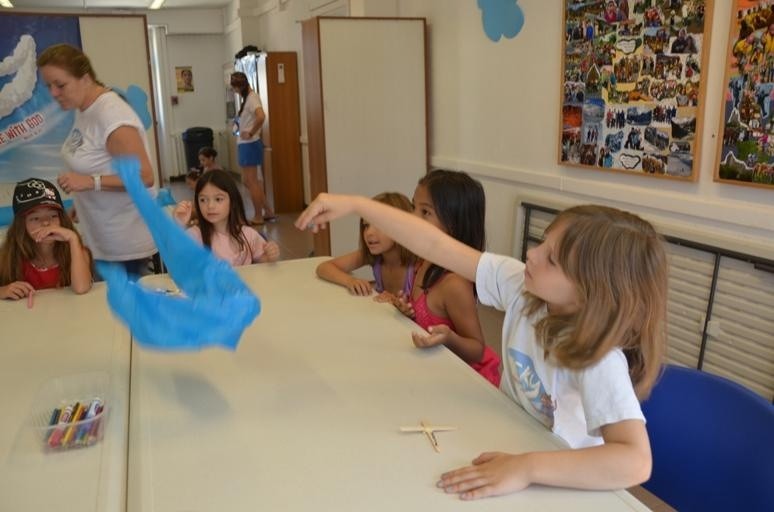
263,215,280,221
247,220,265,227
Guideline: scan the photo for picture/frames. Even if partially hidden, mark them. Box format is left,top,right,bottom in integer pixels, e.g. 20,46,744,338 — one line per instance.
712,0,773,191
557,1,716,184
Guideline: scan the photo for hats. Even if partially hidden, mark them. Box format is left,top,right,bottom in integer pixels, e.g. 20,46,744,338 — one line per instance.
12,178,63,217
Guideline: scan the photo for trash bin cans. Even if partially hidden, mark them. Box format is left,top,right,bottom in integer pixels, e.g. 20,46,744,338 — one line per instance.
182,127,214,170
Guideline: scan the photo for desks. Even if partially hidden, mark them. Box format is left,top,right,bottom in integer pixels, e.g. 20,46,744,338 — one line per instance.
127,255,657,512
1,278,131,512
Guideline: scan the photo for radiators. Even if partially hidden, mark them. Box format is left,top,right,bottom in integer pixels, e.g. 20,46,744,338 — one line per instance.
172,131,221,178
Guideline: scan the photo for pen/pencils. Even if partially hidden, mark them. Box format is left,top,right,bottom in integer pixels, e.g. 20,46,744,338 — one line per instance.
43,397,106,446
28,290,33,308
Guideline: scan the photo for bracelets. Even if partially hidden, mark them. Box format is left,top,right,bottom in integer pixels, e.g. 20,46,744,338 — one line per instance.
247,132,252,137
90,171,103,194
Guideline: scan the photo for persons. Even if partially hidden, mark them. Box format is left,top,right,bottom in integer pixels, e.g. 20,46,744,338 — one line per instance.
185,144,226,187
0,178,94,303
560,2,705,172
228,71,275,224
294,192,671,501
395,170,497,378
33,41,159,282
314,191,410,299
178,68,193,92
722,3,773,182
170,170,283,265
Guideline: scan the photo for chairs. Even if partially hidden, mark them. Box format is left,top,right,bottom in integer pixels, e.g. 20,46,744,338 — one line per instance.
638,364,773,511
